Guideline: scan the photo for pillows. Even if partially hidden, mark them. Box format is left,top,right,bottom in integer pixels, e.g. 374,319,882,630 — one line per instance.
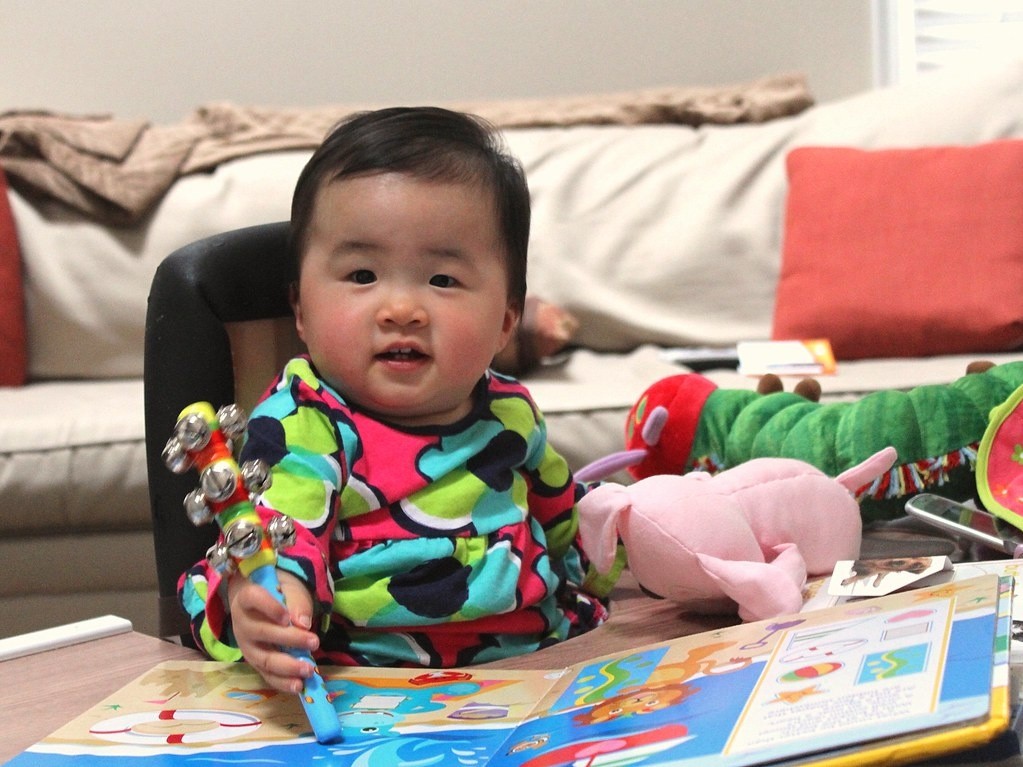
0,167,27,386
771,139,1023,359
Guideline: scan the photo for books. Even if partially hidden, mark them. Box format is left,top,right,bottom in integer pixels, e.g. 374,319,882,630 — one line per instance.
0,576,1015,767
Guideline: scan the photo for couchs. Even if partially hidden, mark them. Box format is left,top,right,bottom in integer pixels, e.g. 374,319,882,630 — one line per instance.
0,84,1023,639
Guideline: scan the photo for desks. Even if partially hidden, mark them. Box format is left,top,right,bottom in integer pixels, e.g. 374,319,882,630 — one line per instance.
0,565,744,767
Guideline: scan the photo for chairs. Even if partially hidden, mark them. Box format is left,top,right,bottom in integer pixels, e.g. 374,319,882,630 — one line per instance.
144,220,306,653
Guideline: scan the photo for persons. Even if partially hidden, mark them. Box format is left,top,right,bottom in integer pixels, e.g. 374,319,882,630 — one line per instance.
179,106,611,695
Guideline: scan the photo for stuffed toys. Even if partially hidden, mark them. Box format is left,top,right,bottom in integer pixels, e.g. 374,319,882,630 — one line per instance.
580,446,898,622
573,359,1023,521
